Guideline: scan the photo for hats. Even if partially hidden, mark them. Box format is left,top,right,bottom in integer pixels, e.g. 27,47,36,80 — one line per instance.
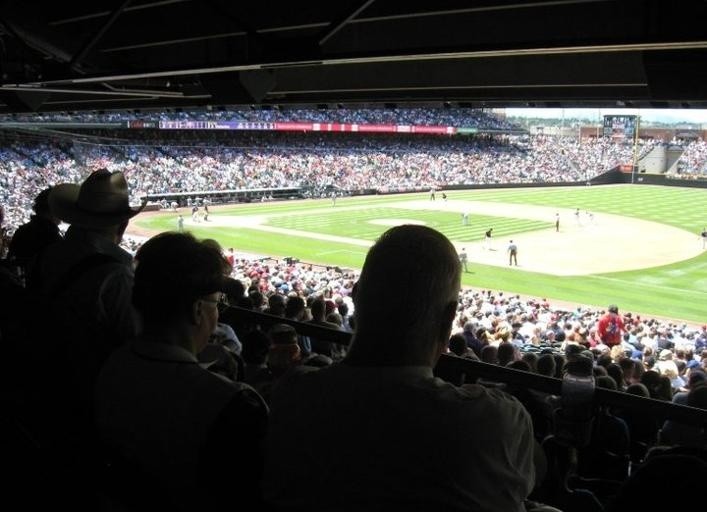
46,168,147,230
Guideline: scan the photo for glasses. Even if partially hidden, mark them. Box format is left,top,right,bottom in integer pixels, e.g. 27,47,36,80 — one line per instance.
200,292,229,313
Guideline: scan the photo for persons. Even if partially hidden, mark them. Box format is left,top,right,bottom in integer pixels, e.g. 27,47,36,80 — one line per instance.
0,104,706,511
